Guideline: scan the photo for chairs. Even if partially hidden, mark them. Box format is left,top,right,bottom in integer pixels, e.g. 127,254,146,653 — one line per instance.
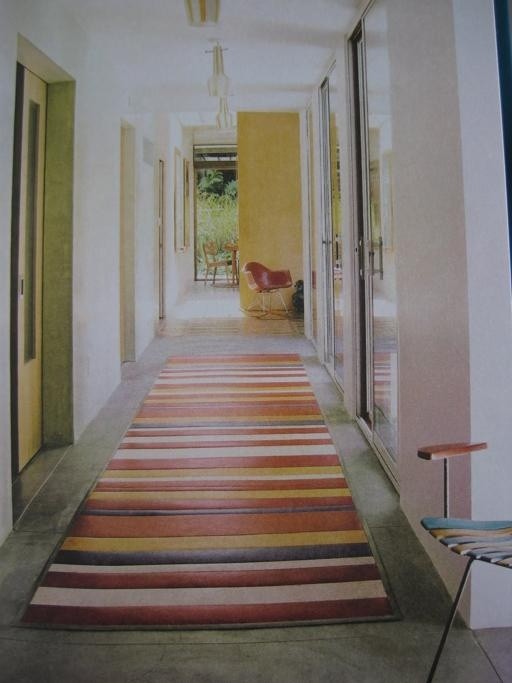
241,260,293,319
202,239,239,287
416,440,512,683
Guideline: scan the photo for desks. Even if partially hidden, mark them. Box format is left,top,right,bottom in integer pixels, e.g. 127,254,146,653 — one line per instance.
222,245,240,288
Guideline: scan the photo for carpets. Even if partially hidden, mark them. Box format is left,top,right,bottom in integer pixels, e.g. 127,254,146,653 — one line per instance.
10,352,406,631
309,315,397,424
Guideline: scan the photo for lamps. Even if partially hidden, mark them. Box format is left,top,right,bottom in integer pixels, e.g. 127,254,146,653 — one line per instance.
183,0,234,131
